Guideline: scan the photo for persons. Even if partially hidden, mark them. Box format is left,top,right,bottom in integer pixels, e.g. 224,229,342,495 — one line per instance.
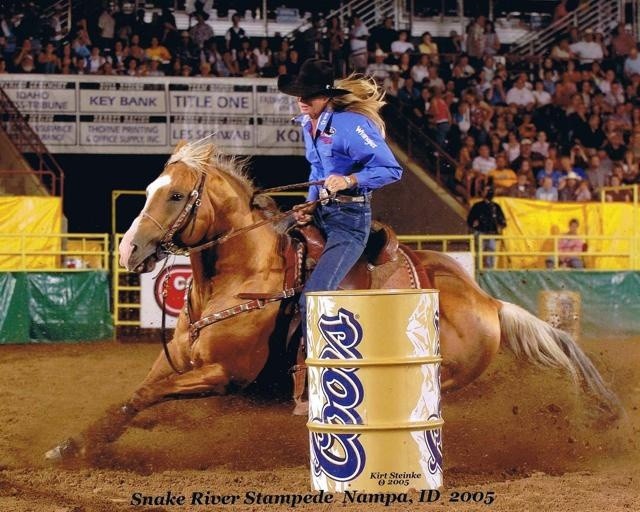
293,96,403,354
546,219,583,268
1,0,640,202
467,184,506,268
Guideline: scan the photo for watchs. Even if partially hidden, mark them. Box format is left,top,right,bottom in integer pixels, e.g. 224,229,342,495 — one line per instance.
343,176,352,188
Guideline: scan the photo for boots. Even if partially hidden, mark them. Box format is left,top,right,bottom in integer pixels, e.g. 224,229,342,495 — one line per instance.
294,337,309,415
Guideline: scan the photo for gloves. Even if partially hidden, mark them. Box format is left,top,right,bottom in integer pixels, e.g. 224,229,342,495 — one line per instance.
325,175,357,192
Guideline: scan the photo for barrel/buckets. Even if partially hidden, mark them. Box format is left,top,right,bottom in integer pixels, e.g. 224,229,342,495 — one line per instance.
303,290,442,493
538,289,580,340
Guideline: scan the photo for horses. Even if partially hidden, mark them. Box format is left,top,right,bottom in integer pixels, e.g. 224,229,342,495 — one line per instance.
44,132,628,466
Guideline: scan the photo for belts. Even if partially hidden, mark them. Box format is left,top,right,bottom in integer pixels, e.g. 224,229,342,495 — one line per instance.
318,189,366,205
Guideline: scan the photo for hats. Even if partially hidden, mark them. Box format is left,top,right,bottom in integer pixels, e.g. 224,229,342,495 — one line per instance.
278,58,353,97
521,139,531,144
564,172,581,181
